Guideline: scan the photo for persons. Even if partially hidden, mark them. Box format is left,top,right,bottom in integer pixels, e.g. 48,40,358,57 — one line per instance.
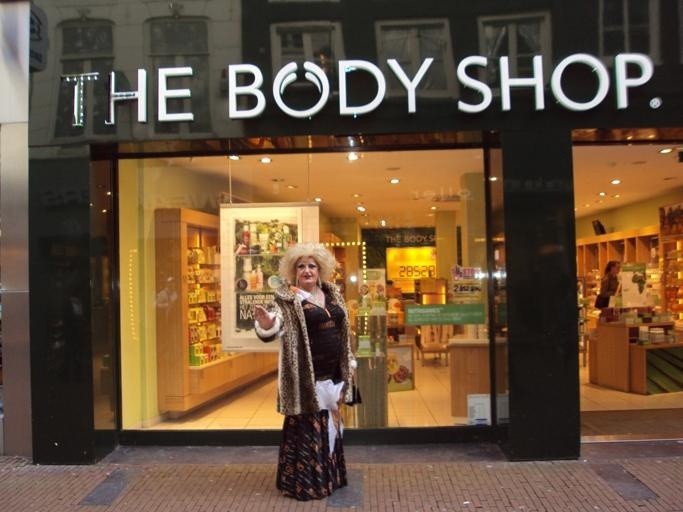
251,244,359,503
598,258,622,311
155,275,178,314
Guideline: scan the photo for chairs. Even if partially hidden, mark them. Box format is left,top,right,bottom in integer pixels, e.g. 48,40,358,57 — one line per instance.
413,323,451,366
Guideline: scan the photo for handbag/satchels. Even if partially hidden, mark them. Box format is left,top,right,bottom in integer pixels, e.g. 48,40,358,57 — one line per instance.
595,295,610,310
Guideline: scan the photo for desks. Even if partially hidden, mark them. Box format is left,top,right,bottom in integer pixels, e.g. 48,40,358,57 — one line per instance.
588,321,683,396
446,333,491,417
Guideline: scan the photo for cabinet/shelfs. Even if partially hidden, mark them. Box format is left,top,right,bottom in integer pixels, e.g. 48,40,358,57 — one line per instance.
153,206,278,420
319,232,346,303
576,222,683,333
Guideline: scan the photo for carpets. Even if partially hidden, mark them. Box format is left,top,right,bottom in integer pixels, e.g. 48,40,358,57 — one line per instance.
580,407,682,435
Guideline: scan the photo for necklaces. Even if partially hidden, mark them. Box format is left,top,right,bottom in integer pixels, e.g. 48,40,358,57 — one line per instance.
307,289,323,309
